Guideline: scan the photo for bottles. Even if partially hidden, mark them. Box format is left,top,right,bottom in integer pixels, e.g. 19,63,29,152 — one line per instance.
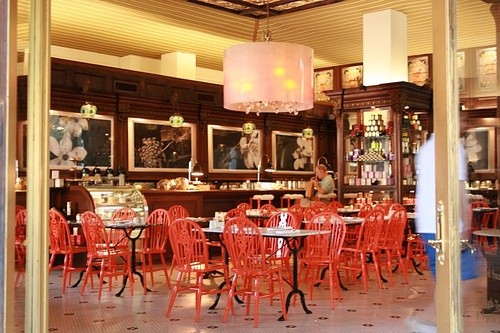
275,177,304,190
212,179,254,190
209,215,223,229
60,200,83,247
81,164,90,185
106,164,113,184
93,164,102,184
118,166,125,186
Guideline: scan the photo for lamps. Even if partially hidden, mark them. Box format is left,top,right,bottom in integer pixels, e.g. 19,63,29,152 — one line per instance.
188,156,205,189
255,153,275,190
222,0,313,117
317,156,335,173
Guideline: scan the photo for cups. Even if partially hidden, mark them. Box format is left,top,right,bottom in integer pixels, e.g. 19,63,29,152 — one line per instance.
471,179,493,189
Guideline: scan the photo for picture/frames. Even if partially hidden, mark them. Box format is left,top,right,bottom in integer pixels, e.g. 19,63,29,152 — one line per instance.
313,66,337,106
337,62,363,89
127,117,196,172
271,130,318,175
457,44,500,98
407,53,433,89
460,125,497,181
48,110,115,171
17,120,28,173
207,124,263,174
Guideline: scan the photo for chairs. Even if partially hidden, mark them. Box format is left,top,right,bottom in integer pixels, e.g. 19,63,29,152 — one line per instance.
465,201,492,262
13,200,428,328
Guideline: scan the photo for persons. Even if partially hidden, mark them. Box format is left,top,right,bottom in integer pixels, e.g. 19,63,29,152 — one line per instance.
262,154,274,169
408,106,479,333
310,164,334,202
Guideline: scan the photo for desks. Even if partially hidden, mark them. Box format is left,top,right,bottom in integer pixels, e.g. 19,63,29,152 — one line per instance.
188,226,331,321
472,207,500,314
66,216,165,297
301,215,391,291
245,207,361,268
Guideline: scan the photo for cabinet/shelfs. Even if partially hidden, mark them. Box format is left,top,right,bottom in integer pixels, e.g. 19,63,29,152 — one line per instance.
322,82,433,206
60,184,149,270
